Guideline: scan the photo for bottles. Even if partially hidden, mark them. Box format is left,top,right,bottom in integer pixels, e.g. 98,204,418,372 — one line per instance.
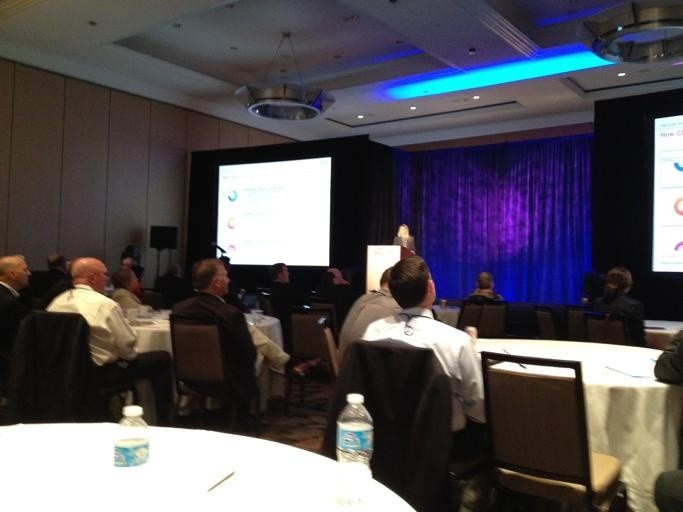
113,405,150,511
253,299,263,325
336,393,373,505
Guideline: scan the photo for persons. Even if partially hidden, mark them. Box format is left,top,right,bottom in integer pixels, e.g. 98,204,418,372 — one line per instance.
219,255,252,312
44,257,176,427
322,265,350,332
652,325,683,512
45,252,75,304
109,252,155,317
338,265,408,371
392,224,416,254
169,259,270,427
590,267,646,352
453,271,508,329
1,255,33,401
268,263,312,319
358,255,489,467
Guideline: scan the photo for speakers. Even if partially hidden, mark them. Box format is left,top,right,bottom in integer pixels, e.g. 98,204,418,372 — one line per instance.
150,225,178,248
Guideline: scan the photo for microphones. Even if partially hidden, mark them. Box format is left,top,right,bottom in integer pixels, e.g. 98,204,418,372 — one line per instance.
210,241,225,254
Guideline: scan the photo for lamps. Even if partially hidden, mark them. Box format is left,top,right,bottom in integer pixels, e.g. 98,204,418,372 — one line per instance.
233,33,335,121
574,2,681,68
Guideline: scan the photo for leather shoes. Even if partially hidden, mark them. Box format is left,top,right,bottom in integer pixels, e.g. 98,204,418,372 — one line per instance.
291,357,323,377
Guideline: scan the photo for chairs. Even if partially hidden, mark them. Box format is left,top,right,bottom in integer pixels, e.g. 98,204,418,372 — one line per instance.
0,269,681,511
